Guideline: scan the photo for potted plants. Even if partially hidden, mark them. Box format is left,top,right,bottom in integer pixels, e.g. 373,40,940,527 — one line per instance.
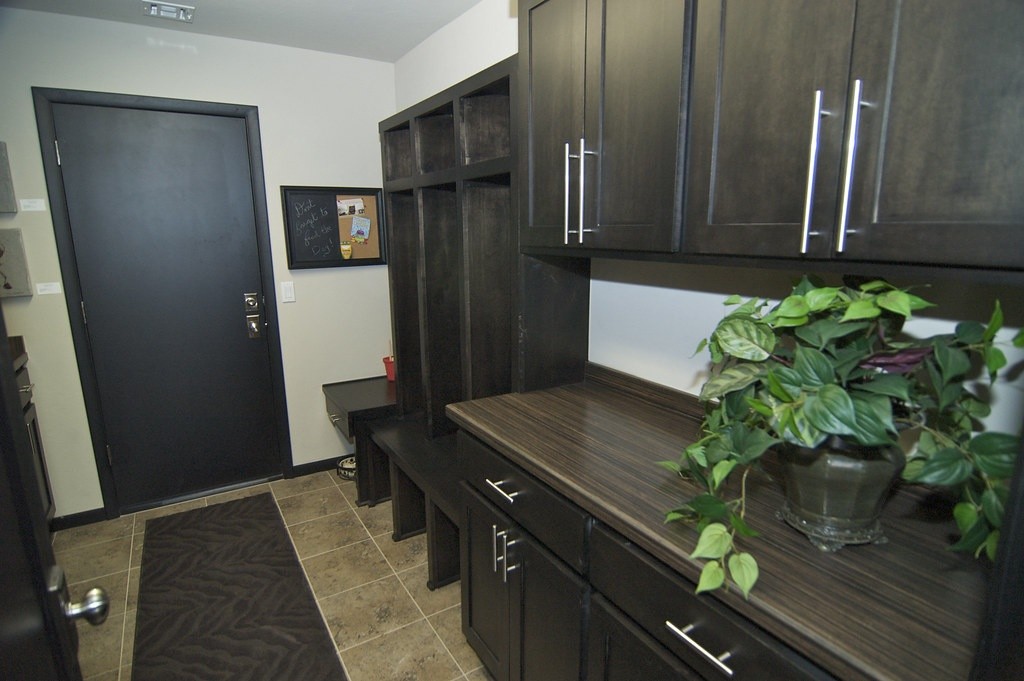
646,266,1024,609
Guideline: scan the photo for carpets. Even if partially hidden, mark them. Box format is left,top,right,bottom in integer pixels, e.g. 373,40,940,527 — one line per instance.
130,492,350,681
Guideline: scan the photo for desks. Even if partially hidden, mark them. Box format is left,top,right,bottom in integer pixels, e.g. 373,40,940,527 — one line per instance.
443,379,993,681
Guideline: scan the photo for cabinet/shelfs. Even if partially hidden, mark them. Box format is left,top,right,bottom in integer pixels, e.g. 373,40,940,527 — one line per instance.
12,352,57,526
517,0,692,264
322,384,355,444
680,0,1023,275
454,426,835,681
377,54,522,440
